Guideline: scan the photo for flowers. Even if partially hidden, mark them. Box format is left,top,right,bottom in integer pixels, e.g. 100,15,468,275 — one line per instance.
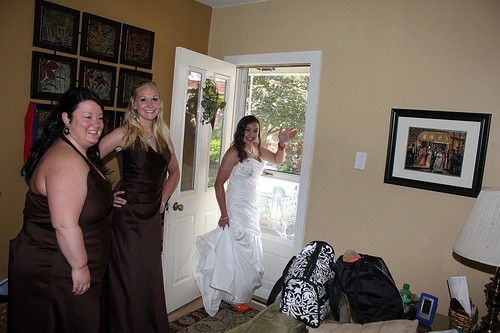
201,78,226,124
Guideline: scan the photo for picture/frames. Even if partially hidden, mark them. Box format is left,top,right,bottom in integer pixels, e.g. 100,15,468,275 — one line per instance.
78,60,116,107
80,12,121,64
96,110,126,146
384,108,492,199
120,23,155,69
30,51,78,101
416,293,438,326
117,67,153,108
33,0,80,55
36,103,60,151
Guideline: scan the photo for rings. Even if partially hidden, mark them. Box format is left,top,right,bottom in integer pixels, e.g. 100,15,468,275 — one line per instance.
77,287,82,292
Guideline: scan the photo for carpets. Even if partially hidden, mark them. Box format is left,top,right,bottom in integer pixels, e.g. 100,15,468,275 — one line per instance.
168,299,261,333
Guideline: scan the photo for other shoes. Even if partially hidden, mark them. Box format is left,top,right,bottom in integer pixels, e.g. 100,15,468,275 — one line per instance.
233,304,248,311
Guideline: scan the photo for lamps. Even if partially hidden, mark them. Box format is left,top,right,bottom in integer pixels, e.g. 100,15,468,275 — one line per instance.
453,187,500,333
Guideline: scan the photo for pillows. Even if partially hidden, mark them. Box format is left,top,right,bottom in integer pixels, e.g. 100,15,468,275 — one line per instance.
306,320,419,333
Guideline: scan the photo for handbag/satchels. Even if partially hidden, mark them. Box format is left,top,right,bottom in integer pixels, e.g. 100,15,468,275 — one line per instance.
328,251,405,323
266,241,336,328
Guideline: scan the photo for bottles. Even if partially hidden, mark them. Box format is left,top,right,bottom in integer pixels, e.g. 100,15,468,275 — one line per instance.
400,284,412,319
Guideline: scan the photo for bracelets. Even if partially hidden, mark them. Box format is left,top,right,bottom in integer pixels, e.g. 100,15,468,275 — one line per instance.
219,217,229,220
277,142,286,151
161,202,165,206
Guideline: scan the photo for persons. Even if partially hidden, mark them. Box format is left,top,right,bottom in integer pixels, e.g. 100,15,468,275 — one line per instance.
97,79,182,333
6,87,114,333
191,114,298,317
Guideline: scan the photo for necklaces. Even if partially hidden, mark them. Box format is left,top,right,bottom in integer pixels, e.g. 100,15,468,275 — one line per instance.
243,144,254,155
